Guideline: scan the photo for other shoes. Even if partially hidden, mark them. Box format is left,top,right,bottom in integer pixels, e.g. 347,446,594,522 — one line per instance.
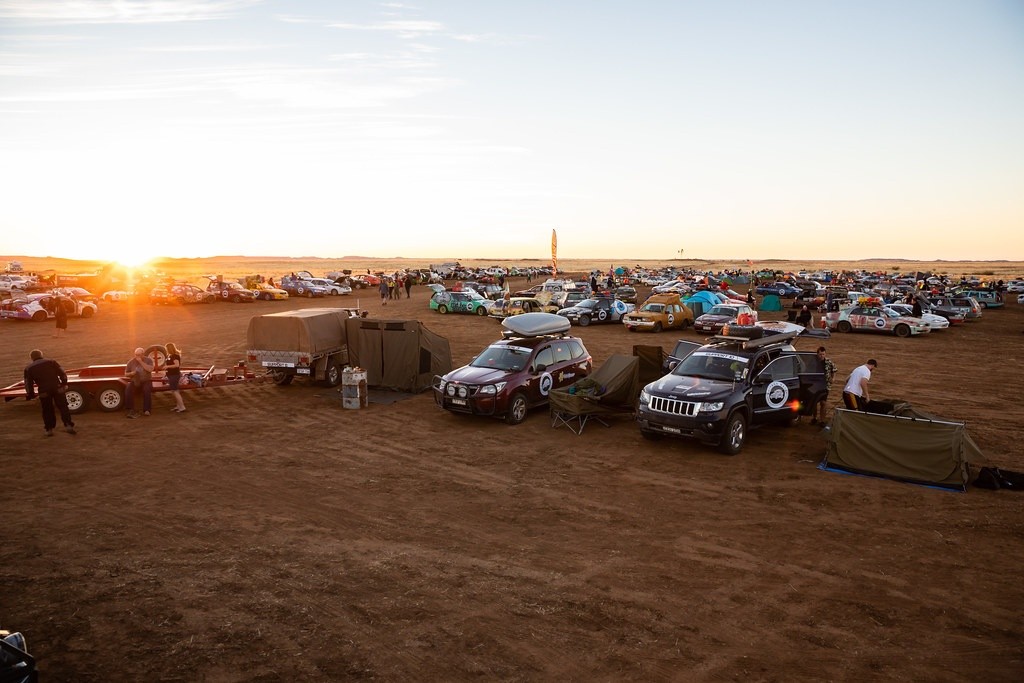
145,411,150,415
820,422,826,428
127,410,139,418
176,410,185,413
44,429,52,436
171,407,178,411
66,425,74,433
811,419,817,425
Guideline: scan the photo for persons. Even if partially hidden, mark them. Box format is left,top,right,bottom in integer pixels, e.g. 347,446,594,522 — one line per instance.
24,349,75,436
53,297,68,338
123,347,154,418
162,343,186,413
498,275,504,287
808,347,837,428
590,272,613,292
909,298,922,318
843,359,878,410
379,276,411,305
268,278,274,286
796,305,811,327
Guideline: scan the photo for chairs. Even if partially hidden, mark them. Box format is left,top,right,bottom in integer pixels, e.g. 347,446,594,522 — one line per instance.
547,351,640,436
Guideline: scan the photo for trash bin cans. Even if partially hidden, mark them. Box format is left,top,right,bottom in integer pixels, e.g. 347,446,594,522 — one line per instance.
343,369,368,410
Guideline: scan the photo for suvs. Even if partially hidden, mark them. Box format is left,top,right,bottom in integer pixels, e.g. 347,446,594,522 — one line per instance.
430,310,595,427
632,317,830,458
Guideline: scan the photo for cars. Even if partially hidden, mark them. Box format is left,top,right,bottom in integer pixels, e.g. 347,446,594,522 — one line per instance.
0,266,1024,338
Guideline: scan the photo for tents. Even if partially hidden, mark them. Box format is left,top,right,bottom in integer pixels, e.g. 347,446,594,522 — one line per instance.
345,317,452,395
688,290,722,313
